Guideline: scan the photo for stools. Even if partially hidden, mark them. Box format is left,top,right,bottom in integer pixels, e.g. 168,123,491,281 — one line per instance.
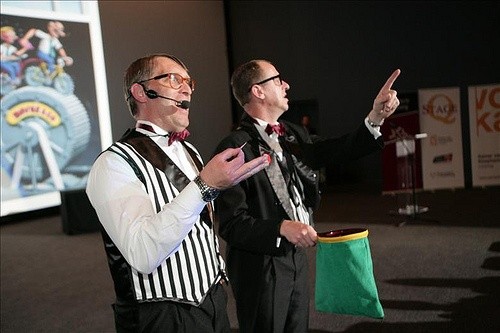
61,189,102,235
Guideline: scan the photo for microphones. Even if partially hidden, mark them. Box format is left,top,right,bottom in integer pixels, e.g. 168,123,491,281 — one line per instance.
146,89,191,109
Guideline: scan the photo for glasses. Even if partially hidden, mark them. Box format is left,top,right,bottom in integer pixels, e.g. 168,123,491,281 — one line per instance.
137,73,195,92
247,73,282,92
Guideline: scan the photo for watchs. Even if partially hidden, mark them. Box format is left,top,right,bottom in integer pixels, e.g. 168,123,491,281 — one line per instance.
194,176,220,202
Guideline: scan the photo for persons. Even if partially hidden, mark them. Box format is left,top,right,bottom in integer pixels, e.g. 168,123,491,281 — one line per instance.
86,53,269,333
211,58,401,333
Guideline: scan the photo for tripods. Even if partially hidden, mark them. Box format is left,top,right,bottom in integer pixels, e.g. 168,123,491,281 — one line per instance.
388,122,442,227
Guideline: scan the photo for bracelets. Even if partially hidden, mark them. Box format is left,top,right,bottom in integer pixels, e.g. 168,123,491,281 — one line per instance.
368,119,382,127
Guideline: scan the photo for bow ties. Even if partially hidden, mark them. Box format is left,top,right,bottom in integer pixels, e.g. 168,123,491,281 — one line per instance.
136,123,191,146
253,119,287,137
